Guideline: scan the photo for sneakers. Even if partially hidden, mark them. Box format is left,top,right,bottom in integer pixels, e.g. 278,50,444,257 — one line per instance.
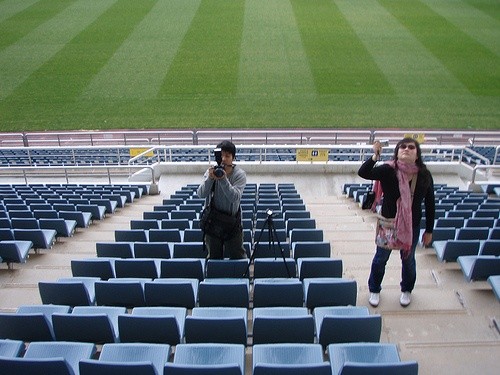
400,292,412,307
368,292,379,307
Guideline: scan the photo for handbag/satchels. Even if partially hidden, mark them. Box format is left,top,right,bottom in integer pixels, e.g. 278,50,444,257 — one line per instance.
376,215,407,250
200,206,240,240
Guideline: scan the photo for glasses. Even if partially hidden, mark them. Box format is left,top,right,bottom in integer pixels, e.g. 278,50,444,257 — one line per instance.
398,145,417,151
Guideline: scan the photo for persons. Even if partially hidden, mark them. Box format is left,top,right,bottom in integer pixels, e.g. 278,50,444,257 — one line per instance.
196,142,253,292
358,138,434,307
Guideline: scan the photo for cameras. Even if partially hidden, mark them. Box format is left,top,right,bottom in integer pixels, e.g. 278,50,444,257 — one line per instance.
266,208,273,216
212,148,225,178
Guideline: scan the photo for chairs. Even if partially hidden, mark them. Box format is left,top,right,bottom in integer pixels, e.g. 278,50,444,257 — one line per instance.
0,147,500,374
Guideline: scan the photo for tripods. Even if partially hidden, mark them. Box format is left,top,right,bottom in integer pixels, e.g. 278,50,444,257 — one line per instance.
244,215,292,278
